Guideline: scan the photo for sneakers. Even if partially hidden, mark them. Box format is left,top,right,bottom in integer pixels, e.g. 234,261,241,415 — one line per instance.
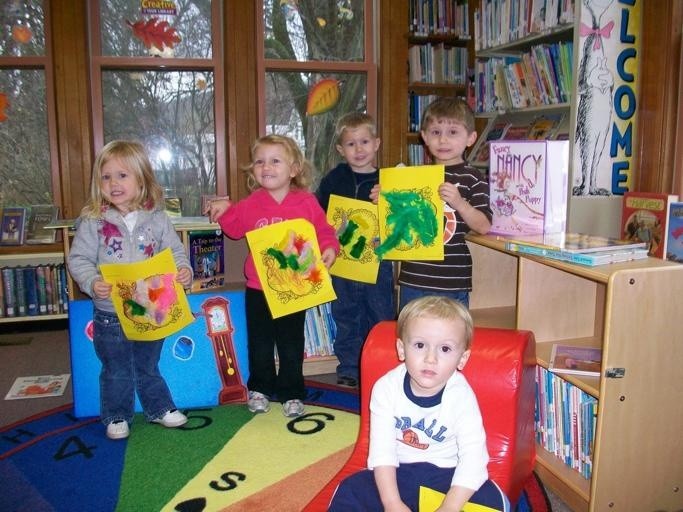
105,418,130,440
281,397,305,418
148,408,190,428
336,376,358,389
245,390,269,414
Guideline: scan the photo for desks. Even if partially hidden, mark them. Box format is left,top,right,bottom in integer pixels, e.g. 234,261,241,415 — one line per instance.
44,216,224,301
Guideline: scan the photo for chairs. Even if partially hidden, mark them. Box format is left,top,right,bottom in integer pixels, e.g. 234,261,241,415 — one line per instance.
302,321,536,512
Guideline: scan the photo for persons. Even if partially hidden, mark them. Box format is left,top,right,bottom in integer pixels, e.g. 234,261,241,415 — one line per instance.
369,98,492,321
314,113,397,391
327,296,510,512
67,139,194,440
204,135,340,417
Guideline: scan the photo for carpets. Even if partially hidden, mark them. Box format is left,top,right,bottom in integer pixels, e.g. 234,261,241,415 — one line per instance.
0,378,552,511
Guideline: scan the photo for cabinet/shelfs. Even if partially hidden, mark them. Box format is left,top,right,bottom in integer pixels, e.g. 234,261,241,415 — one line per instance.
390,1,473,170
464,234,682,512
0,245,69,322
474,0,642,238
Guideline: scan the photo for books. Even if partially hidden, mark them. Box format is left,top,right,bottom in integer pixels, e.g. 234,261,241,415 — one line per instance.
188,230,225,294
534,344,601,480
407,0,575,168
273,302,338,365
496,192,683,267
0,205,76,318
